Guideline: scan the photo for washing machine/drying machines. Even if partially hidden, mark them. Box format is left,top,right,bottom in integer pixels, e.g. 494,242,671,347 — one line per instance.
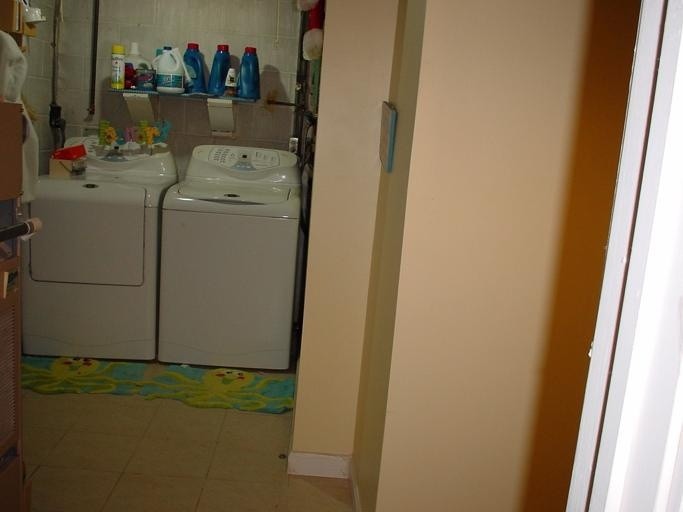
21,134,178,361
158,145,301,369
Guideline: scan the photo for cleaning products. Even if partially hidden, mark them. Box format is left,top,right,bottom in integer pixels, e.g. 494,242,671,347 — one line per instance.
238,46,261,98
152,47,185,94
209,44,232,95
126,42,152,71
184,43,208,94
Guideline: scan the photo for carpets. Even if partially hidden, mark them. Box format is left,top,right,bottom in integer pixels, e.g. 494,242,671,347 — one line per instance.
20,355,295,414
